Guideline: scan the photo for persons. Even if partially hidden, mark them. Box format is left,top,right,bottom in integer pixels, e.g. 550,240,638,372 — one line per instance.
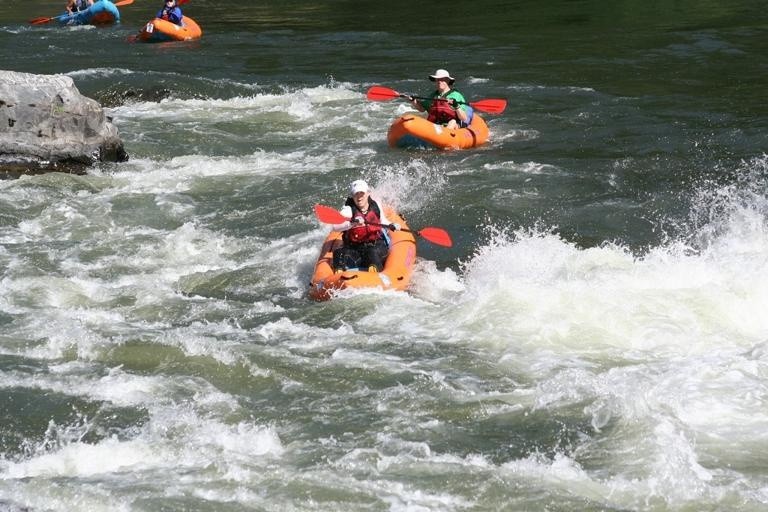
157,0,182,26
333,180,390,274
404,69,467,129
66,0,94,16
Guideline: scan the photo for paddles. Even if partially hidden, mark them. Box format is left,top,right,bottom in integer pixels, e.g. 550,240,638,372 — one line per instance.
43,0,134,24
367,86,507,114
126,0,191,43
314,204,452,247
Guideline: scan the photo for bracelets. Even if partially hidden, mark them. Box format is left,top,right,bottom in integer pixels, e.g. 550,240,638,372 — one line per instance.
454,106,460,110
410,98,415,101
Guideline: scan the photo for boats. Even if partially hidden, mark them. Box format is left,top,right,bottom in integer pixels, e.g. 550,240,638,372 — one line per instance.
308,203,420,297
140,12,203,41
387,109,489,151
58,0,121,25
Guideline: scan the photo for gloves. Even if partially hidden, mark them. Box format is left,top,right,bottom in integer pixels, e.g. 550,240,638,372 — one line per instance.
389,223,401,232
353,216,364,226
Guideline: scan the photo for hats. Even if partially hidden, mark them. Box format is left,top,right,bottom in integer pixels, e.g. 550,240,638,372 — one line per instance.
349,179,369,196
429,69,455,85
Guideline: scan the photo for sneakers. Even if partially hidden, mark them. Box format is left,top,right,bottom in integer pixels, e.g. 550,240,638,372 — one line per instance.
334,267,344,274
368,264,380,272
